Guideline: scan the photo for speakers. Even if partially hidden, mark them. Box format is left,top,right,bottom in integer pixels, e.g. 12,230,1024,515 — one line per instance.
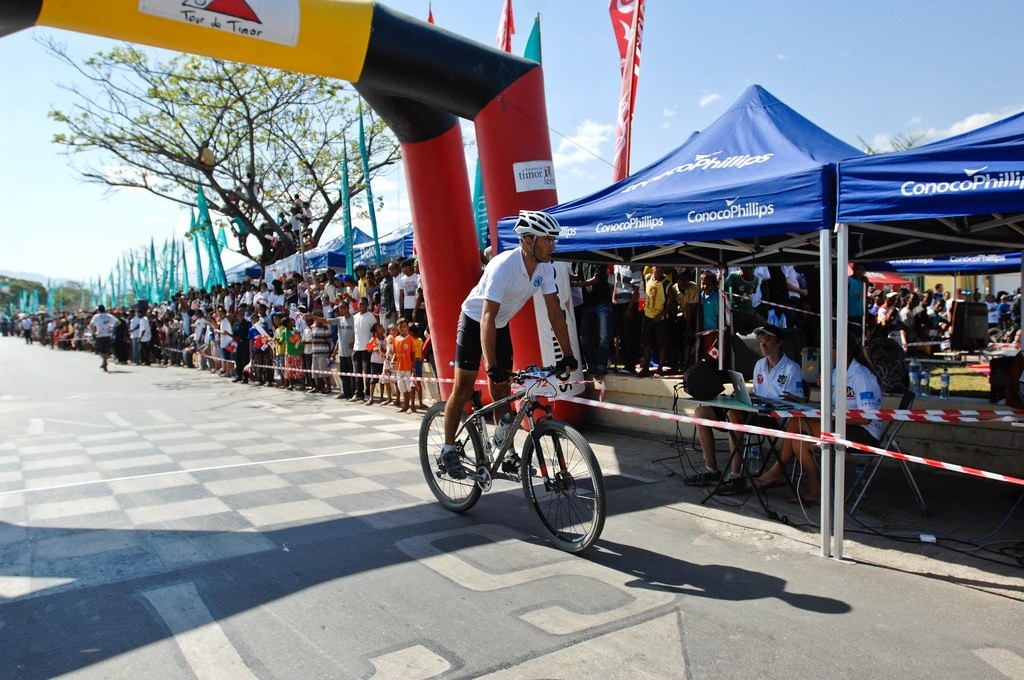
683,361,726,401
950,301,989,351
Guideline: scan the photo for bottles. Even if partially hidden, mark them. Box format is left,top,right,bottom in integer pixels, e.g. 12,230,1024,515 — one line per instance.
908,356,921,398
939,369,949,400
919,367,930,401
749,445,759,473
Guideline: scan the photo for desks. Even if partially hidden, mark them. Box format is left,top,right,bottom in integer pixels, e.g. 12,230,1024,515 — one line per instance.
687,396,835,517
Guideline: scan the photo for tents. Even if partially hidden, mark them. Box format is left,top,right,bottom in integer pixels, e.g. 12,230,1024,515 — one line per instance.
496,85,869,556
835,112,1024,560
223,223,414,285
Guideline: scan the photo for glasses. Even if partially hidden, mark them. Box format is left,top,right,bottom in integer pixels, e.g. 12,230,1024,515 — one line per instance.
536,238,559,246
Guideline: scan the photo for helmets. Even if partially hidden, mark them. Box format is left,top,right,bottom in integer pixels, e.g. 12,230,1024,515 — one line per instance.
353,261,367,271
342,275,354,283
334,273,343,280
513,210,561,236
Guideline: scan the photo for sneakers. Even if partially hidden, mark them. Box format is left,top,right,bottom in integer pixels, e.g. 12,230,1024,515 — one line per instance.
502,453,537,477
440,448,465,478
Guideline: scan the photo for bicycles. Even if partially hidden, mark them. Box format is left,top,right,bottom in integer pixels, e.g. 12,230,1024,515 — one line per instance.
419,361,606,553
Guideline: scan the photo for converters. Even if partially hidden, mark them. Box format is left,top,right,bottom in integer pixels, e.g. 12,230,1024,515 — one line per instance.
919,534,936,543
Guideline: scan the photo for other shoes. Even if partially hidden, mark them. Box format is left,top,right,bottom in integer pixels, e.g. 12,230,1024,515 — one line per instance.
684,470,720,485
212,367,427,414
721,474,745,495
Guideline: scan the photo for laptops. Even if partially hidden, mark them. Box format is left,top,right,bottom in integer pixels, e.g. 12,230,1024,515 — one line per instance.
728,369,788,407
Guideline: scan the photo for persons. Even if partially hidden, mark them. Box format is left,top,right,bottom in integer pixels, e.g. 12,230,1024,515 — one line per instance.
0,256,441,414
569,264,1024,506
233,174,314,260
439,211,579,480
195,141,216,166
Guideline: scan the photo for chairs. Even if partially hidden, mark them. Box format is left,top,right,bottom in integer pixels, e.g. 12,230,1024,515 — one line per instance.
845,390,930,515
752,378,810,483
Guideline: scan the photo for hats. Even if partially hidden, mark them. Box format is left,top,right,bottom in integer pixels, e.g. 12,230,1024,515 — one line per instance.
270,310,282,317
754,325,783,338
412,259,418,267
